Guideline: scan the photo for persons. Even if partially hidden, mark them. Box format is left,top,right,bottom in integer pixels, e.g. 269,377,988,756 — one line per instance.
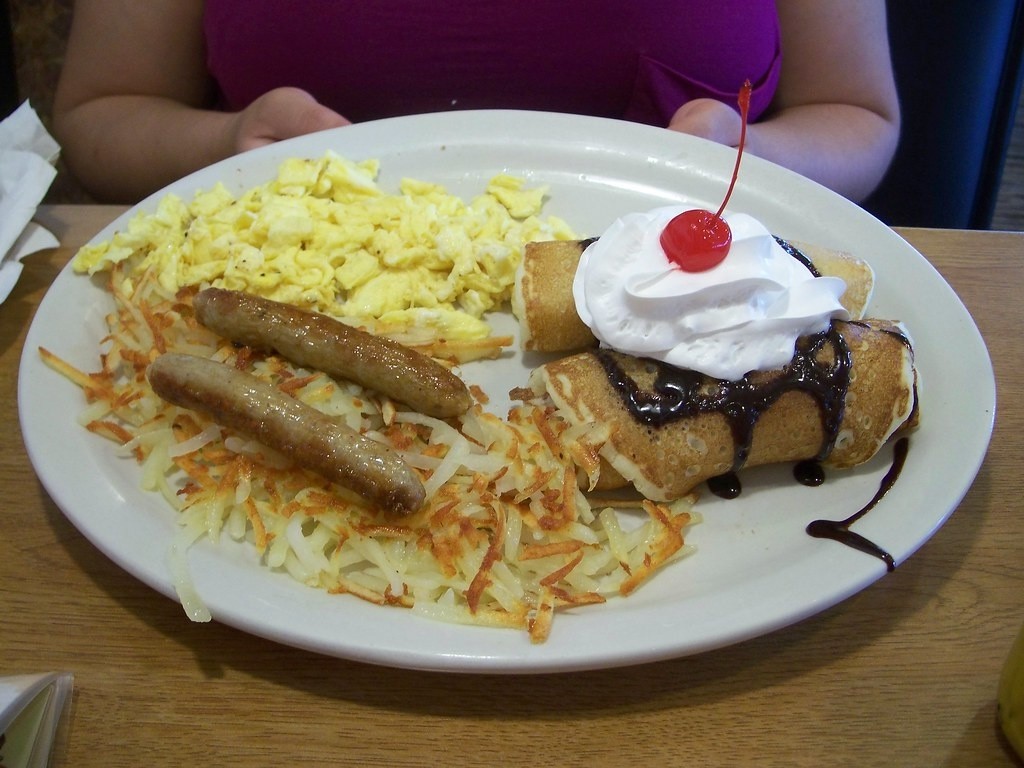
53,0,900,207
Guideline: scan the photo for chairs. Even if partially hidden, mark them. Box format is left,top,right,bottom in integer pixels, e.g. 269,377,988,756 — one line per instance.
858,0,1024,231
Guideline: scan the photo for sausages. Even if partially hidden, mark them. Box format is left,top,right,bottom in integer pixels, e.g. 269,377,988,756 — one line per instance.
191,285,472,416
144,349,427,516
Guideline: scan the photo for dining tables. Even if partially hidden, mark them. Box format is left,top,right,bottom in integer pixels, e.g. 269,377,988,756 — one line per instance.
0,206,1020,768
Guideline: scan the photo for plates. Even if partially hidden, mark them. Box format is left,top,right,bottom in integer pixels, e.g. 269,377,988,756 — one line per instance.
15,107,999,680
